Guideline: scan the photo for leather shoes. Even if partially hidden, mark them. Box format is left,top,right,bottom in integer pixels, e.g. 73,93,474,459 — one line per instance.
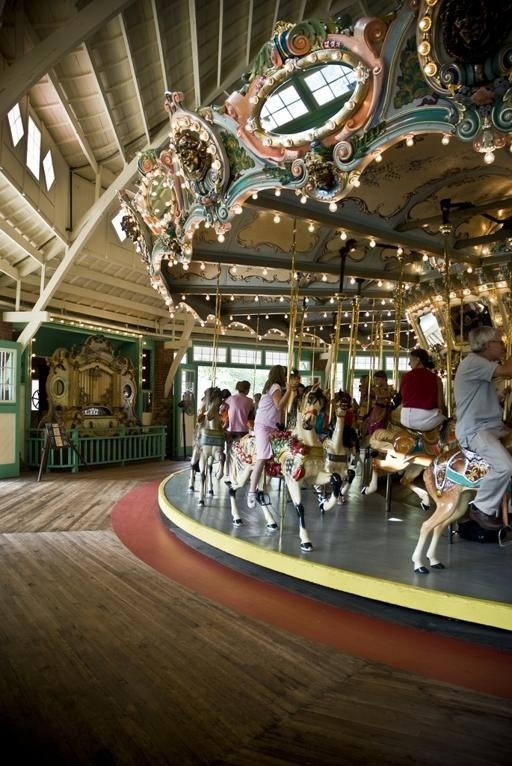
469,507,503,531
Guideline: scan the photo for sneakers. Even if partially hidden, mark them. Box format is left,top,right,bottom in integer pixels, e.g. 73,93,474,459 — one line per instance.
247,489,257,508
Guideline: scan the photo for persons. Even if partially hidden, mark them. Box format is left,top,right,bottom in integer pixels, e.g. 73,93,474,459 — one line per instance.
452,323,512,533
193,364,306,509
397,345,450,433
355,371,396,434
